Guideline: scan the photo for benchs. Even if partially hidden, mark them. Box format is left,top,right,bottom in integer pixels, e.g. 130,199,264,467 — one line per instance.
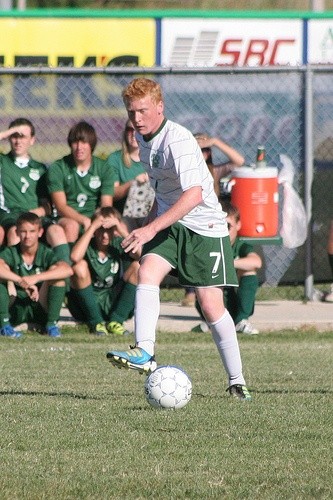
240,235,282,246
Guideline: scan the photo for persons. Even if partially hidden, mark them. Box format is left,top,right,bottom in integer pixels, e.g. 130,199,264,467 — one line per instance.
0,213,76,337
65,207,140,337
46,121,115,308
195,204,266,335
193,133,245,201
107,78,251,402
105,118,157,233
0,118,74,297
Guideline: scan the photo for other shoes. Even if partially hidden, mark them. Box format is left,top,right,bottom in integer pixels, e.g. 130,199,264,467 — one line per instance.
1,325,21,336
90,320,130,337
236,319,259,335
45,325,60,337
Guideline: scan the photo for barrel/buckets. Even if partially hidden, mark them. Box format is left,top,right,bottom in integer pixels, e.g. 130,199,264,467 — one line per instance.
223,166,279,238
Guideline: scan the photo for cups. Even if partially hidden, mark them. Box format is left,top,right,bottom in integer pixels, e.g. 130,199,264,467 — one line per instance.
256,146,266,168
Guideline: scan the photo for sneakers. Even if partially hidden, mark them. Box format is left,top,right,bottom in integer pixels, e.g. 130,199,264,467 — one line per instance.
106,341,158,375
225,384,251,400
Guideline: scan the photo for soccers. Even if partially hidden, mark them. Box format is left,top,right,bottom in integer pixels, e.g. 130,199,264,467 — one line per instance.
145,366,192,409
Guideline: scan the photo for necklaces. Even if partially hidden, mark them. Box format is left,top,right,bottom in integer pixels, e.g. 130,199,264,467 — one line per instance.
15,154,28,167
77,162,93,173
98,253,108,261
23,254,35,268
